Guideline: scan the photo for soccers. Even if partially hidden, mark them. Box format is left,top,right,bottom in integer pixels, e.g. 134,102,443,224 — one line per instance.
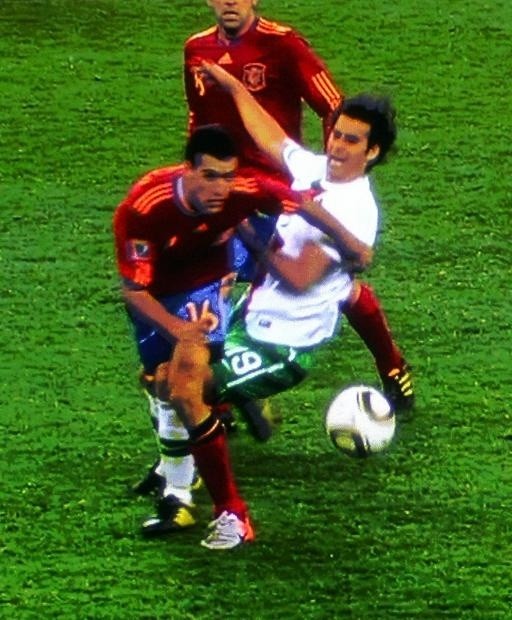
324,385,397,459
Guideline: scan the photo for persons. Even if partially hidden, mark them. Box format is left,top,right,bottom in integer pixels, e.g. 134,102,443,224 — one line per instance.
113,125,376,557
181,0,415,436
130,56,398,538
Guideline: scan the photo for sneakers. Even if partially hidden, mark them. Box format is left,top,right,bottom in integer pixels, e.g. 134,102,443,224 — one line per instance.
132,461,201,535
223,401,273,443
200,508,256,550
381,357,414,411
